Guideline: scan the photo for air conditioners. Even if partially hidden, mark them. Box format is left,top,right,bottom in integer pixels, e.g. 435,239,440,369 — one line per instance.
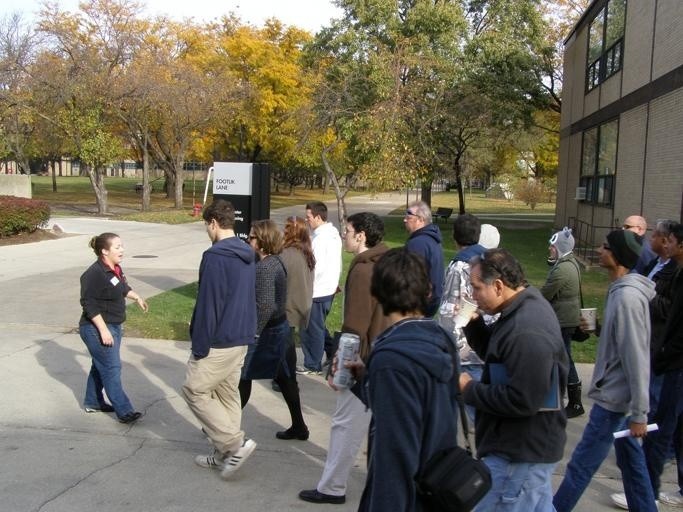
574,186,587,200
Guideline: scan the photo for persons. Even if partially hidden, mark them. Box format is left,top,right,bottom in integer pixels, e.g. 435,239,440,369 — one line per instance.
77,232,150,424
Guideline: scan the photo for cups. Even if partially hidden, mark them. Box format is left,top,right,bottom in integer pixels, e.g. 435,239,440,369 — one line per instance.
579,306,599,332
453,295,478,329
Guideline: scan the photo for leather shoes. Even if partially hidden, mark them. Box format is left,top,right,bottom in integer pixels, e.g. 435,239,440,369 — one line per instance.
84,402,115,413
298,489,346,505
117,411,142,425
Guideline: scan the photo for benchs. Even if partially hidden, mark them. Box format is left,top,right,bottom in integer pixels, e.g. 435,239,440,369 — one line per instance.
431,206,452,223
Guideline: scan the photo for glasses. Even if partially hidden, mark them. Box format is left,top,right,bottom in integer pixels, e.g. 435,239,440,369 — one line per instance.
623,224,639,229
406,211,421,219
248,235,257,241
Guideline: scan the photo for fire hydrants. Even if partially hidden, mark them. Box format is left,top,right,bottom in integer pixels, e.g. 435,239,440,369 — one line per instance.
192,200,203,218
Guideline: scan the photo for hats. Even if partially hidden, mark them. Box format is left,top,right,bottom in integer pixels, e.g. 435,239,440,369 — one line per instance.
549,226,575,258
476,223,502,251
606,229,643,271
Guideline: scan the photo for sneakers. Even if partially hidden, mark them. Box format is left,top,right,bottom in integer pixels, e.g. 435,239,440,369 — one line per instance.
220,437,257,479
296,365,324,376
194,454,224,470
608,491,660,511
275,426,310,441
657,487,683,507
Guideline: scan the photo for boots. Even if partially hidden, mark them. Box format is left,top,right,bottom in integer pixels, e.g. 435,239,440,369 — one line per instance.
565,379,585,419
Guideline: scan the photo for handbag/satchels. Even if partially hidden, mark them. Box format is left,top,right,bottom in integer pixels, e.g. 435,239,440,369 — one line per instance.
571,325,590,343
414,443,495,512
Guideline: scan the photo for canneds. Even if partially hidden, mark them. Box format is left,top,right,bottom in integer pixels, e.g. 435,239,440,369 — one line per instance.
332,333,360,389
